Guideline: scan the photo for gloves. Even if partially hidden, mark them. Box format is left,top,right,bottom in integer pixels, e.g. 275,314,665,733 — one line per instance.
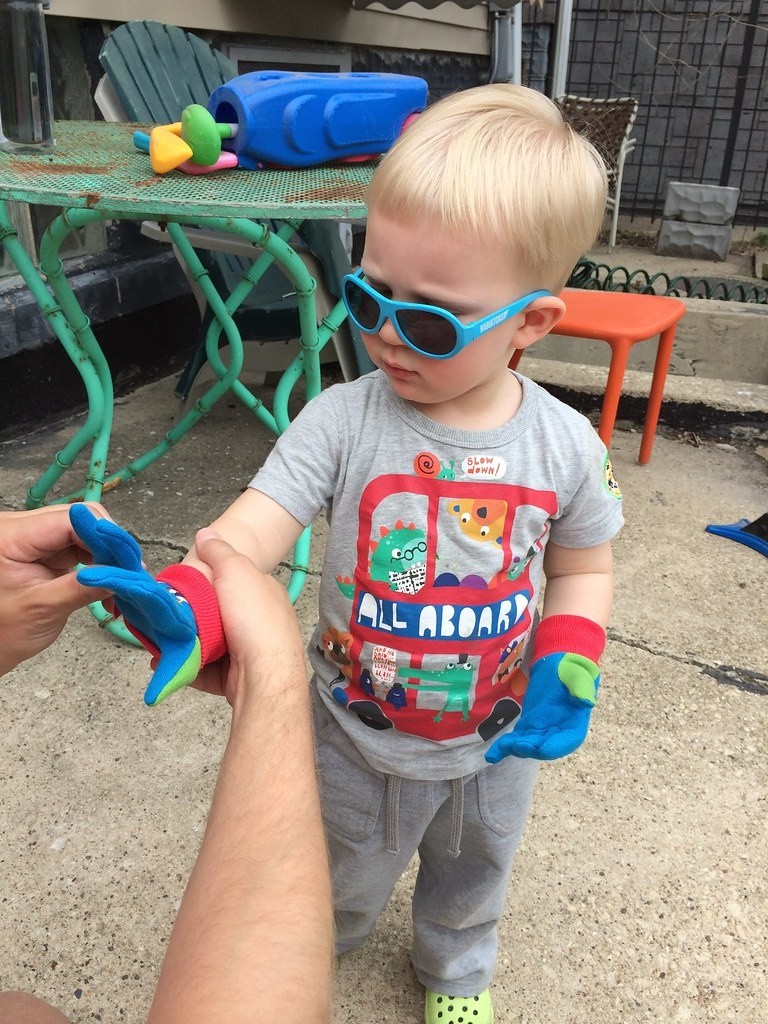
68,504,229,707
484,616,606,765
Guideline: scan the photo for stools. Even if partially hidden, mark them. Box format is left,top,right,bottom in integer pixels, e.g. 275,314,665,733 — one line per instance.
507,286,686,468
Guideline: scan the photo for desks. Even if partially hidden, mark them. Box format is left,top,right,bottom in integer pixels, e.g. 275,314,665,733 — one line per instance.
0,119,385,650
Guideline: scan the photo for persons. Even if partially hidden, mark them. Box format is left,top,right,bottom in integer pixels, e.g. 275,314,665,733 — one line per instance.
0,502,334,1024
69,83,624,1024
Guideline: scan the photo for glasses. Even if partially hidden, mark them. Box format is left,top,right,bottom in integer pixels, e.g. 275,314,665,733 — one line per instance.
340,266,554,359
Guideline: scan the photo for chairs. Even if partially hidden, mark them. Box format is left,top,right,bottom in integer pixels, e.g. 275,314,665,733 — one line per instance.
553,94,638,254
92,20,362,402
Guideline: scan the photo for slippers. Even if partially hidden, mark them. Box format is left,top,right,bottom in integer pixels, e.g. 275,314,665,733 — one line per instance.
424,986,493,1024
